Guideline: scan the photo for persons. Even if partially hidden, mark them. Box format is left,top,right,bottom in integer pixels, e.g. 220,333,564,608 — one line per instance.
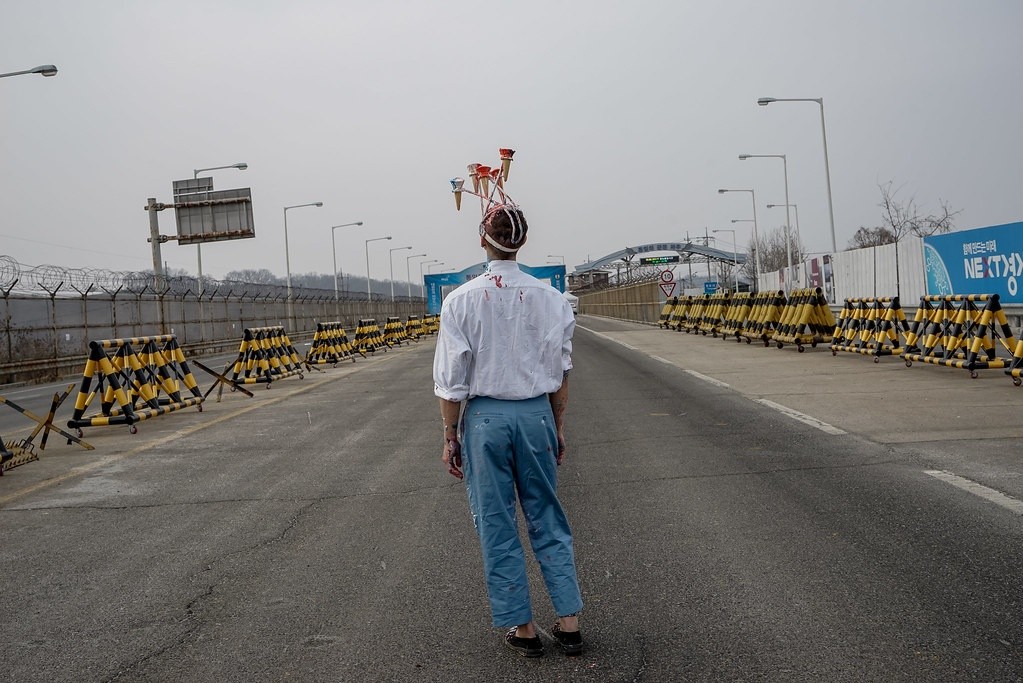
433,208,584,658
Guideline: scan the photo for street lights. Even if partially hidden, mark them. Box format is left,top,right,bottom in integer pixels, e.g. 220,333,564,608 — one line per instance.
406,254,427,316
766,203,803,265
712,229,739,293
756,95,837,253
331,220,364,322
283,200,324,332
428,262,446,275
547,254,564,265
718,187,763,292
388,246,412,317
193,161,248,342
738,153,792,294
365,236,393,317
419,260,439,318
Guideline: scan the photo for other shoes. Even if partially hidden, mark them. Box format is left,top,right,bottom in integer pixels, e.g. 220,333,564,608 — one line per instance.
504,625,545,657
552,620,583,657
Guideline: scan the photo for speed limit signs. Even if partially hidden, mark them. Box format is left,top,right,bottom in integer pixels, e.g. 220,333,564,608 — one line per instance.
660,270,674,282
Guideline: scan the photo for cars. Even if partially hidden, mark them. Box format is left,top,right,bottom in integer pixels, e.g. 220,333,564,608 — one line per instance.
570,303,578,315
